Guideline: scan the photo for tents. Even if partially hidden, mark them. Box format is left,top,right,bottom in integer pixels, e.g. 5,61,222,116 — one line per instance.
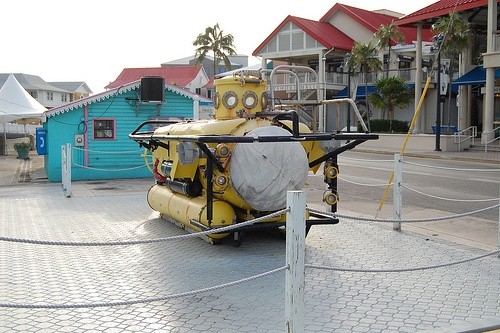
0,73,48,158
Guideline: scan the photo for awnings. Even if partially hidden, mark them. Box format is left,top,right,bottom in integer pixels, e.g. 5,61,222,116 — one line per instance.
331,82,415,99
451,65,500,85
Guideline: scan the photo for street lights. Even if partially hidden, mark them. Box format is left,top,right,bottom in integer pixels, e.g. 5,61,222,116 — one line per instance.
346,52,353,143
429,35,442,152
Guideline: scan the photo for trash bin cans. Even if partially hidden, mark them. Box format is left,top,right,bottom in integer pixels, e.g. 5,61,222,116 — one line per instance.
441,124,450,135
449,125,457,134
430,124,439,134
14,143,32,159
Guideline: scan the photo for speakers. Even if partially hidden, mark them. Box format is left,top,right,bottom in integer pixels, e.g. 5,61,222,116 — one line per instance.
140,75,165,101
